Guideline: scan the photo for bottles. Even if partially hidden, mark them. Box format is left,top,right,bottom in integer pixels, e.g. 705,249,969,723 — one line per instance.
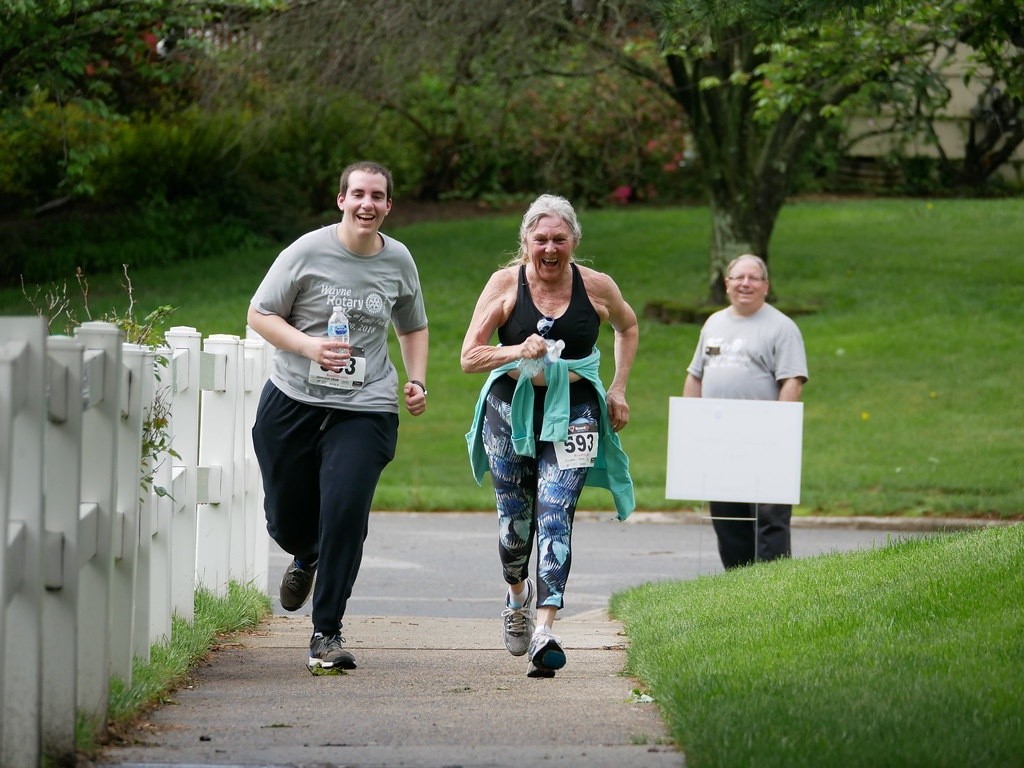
328,306,350,369
516,339,565,379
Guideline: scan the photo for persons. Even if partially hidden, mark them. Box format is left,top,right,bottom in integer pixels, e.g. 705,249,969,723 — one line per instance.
682,257,808,573
246,162,431,676
459,194,640,679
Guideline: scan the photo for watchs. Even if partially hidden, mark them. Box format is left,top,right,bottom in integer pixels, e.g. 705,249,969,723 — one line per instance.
409,380,428,398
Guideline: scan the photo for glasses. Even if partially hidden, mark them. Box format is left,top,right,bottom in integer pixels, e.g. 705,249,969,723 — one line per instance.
728,274,766,285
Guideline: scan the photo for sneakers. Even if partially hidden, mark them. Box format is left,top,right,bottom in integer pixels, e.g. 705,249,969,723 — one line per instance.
308,632,357,670
500,576,536,656
526,631,567,678
279,552,320,612
